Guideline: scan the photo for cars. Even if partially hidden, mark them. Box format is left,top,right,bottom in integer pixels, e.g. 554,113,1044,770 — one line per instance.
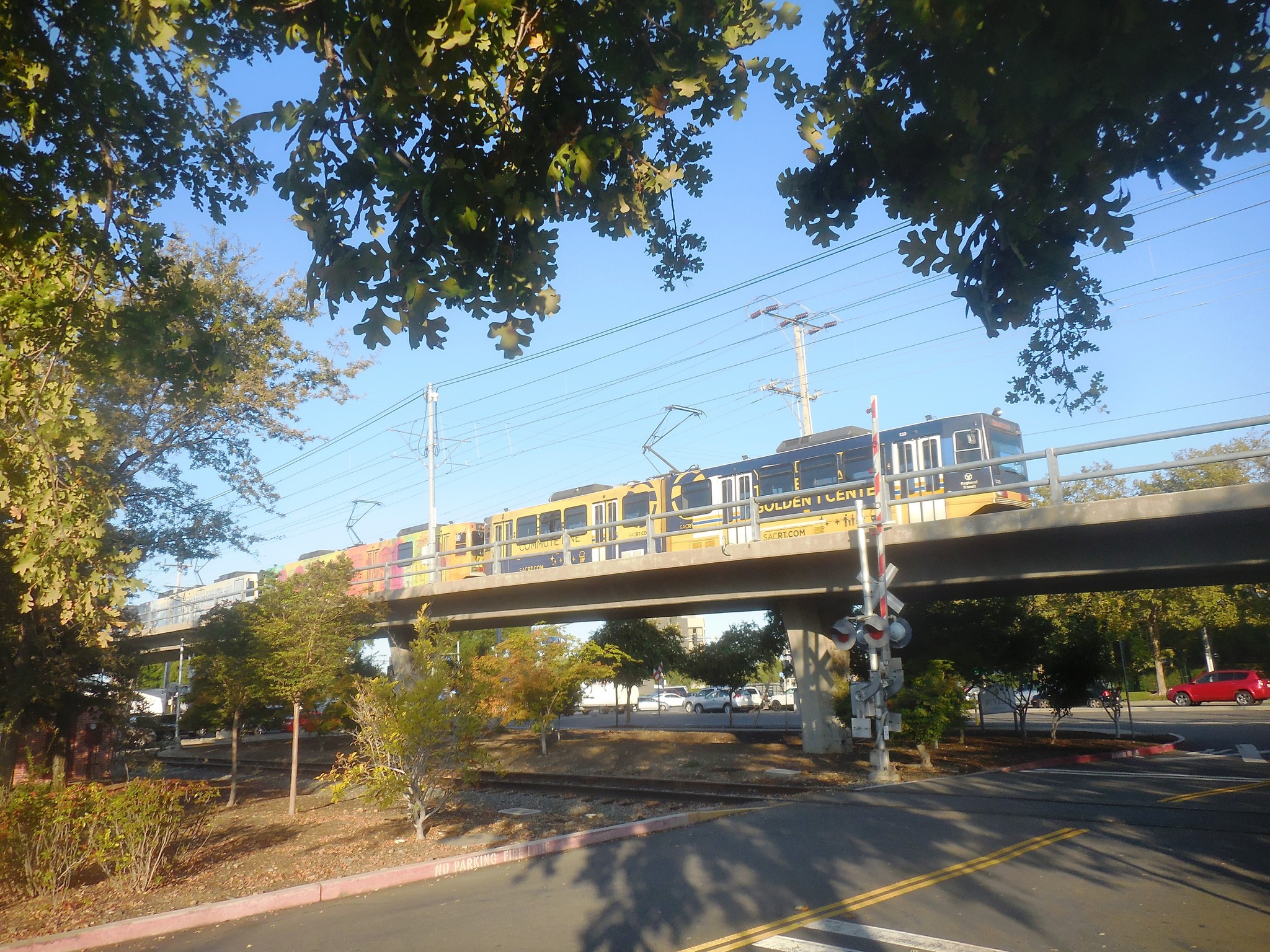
638,678,985,718
1166,669,1270,707
77,673,350,749
1031,680,1122,708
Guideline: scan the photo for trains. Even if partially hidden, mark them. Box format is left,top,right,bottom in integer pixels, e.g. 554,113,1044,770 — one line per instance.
123,408,1032,642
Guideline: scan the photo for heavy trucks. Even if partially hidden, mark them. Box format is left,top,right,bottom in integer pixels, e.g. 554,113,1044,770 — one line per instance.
574,679,640,715
